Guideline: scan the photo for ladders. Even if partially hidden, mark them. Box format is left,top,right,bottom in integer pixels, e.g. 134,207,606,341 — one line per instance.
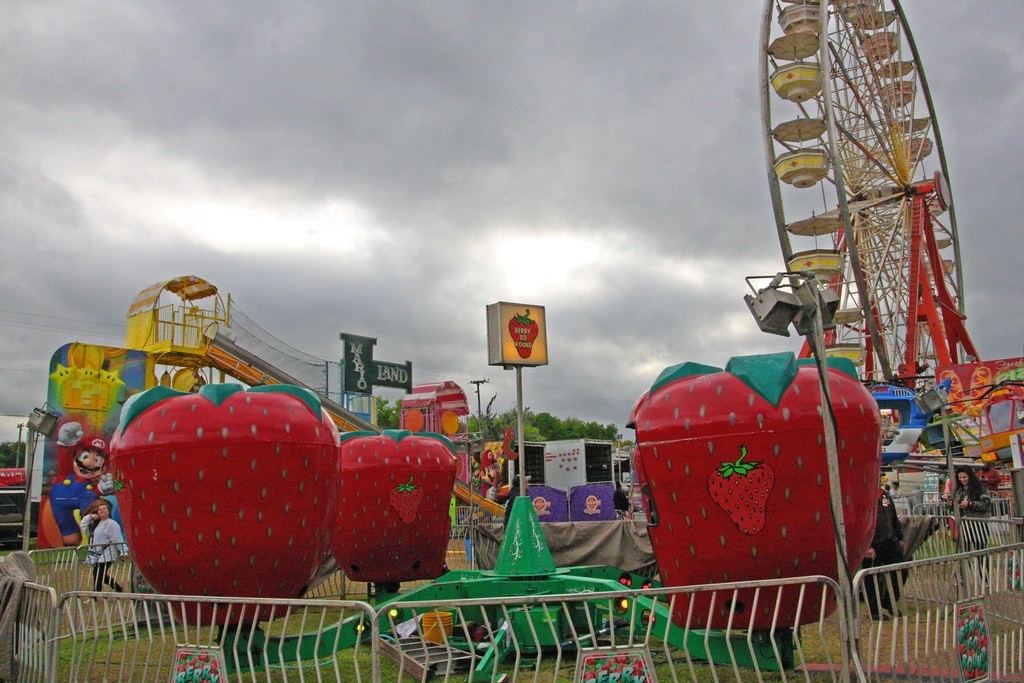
368,632,484,682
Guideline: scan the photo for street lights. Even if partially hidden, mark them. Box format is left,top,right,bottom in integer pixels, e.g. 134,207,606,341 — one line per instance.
16,423,24,468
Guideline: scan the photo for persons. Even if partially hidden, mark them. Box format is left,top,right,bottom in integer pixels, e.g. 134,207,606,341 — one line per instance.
79,502,128,605
504,474,531,534
613,482,640,514
858,465,1001,621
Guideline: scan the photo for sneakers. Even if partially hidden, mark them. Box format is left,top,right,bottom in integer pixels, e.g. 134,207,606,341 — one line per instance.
84,598,97,604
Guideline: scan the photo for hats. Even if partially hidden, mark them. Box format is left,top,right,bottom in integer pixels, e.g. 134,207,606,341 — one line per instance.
512,474,531,485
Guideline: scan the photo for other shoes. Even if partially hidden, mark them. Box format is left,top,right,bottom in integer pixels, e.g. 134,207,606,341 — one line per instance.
867,612,889,621
882,599,903,617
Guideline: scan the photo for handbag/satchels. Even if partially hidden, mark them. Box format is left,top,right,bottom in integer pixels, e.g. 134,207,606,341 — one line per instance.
83,549,102,567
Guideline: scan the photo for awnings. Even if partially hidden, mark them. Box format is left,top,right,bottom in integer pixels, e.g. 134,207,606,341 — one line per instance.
401,381,469,417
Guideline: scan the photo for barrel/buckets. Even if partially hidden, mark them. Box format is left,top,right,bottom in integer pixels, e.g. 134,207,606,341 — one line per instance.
421,612,453,644
